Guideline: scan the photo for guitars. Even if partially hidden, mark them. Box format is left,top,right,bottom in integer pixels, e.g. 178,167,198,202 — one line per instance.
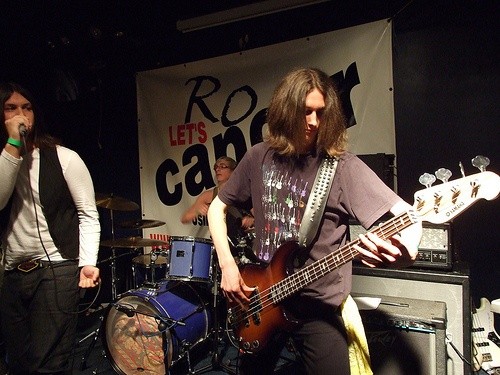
470,296,500,371
226,156,500,356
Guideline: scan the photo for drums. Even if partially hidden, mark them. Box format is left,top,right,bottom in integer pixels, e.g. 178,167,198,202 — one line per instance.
165,235,214,282
130,252,168,289
102,280,208,375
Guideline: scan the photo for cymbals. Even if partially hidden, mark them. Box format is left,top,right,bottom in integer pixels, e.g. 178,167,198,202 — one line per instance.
100,236,170,246
94,196,139,211
119,218,166,230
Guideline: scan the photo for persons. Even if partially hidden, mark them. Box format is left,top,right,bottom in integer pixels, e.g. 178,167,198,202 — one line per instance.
181,157,254,281
207,68,423,375
0,82,102,375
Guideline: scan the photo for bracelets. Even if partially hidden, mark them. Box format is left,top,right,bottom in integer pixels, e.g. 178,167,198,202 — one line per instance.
8,138,23,147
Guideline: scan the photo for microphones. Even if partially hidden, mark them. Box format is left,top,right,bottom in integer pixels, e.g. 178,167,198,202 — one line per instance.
111,303,135,317
19,124,26,136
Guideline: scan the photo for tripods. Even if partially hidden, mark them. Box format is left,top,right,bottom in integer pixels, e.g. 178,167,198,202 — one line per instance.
191,249,240,375
77,208,125,369
